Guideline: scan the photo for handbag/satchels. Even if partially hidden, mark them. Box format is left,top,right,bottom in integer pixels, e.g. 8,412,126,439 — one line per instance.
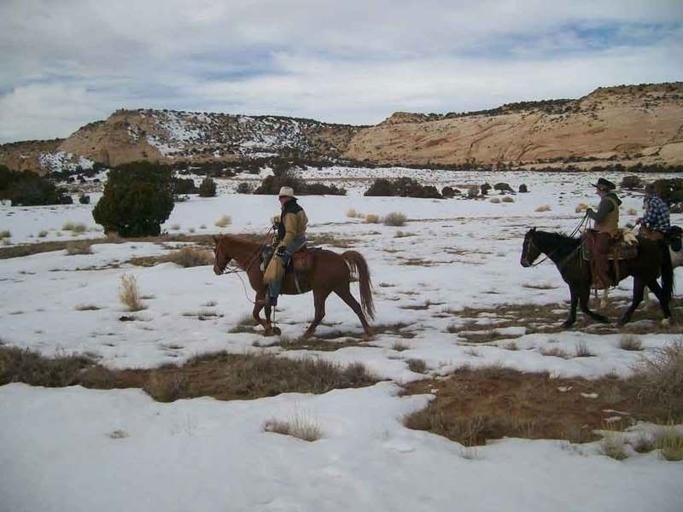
670,225,681,251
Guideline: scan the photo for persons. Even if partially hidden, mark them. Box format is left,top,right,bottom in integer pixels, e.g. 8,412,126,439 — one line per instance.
259,186,308,306
637,184,670,242
585,178,622,289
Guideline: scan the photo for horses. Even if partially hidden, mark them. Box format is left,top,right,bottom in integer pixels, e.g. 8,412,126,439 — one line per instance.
211,232,376,341
520,226,675,330
577,223,683,312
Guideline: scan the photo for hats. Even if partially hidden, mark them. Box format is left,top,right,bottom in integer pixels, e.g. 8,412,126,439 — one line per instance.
591,177,616,191
641,183,656,193
279,186,294,197
